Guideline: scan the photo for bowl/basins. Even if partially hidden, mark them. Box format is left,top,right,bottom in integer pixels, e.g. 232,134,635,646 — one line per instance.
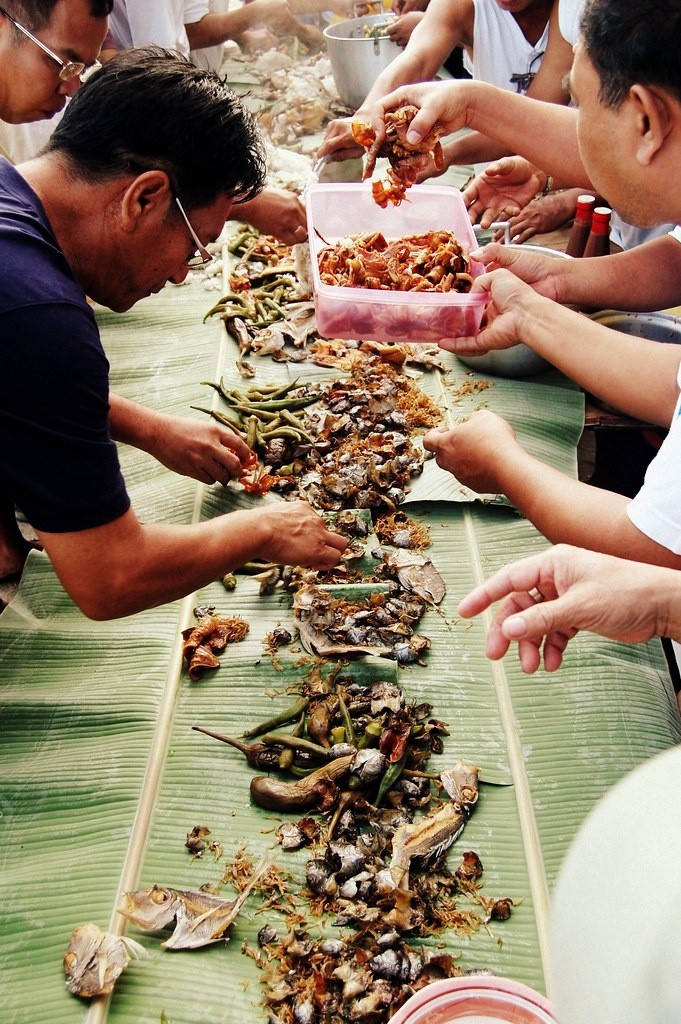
386,977,556,1024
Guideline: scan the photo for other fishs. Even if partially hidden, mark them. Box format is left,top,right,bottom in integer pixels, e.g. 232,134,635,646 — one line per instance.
64,303,514,1024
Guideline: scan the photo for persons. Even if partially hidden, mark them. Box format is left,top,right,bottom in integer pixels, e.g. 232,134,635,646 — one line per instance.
0,0,681,712
0,46,350,622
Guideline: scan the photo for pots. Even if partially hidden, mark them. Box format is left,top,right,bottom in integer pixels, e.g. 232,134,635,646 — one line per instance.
323,0,404,110
588,311,681,347
457,222,574,378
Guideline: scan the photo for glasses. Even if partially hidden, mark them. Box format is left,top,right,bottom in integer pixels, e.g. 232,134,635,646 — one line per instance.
0,7,102,83
527,49,545,81
127,160,212,266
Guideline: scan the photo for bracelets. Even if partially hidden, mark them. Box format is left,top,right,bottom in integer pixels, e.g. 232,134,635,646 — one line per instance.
544,176,553,192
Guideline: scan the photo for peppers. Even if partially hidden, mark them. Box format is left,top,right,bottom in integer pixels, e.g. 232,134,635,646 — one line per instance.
201,222,299,366
190,375,321,452
191,684,423,841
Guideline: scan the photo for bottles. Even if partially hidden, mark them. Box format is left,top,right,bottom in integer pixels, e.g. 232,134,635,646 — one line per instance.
581,207,611,258
565,195,595,258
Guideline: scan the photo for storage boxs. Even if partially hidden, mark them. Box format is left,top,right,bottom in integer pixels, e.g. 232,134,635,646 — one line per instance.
306,183,492,343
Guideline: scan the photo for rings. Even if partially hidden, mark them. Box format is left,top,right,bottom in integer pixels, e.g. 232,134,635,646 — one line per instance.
528,587,544,603
294,225,302,233
